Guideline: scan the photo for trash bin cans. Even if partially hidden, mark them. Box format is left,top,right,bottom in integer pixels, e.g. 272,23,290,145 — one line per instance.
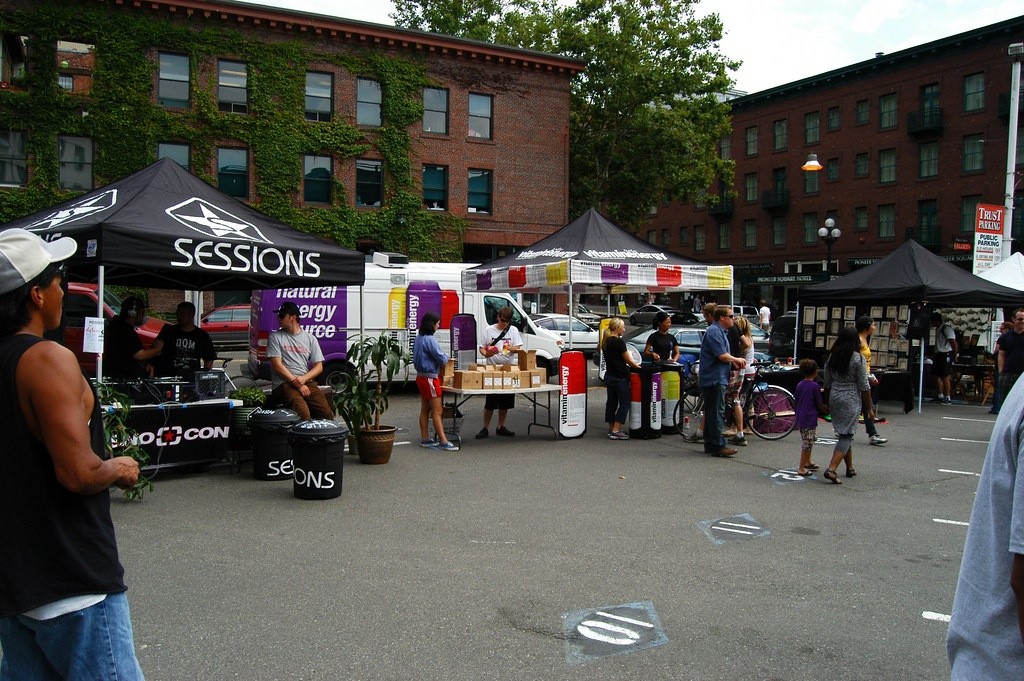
246,409,301,481
287,418,350,500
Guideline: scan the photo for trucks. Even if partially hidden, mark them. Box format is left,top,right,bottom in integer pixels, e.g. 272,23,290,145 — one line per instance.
248,251,566,397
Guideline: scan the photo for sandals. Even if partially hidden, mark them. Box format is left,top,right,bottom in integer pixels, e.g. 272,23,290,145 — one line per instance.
846,468,857,478
824,468,844,484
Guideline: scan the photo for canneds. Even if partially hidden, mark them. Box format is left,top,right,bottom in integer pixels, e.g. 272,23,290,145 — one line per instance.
388,279,459,362
450,313,477,370
558,349,586,439
684,416,690,428
626,360,685,438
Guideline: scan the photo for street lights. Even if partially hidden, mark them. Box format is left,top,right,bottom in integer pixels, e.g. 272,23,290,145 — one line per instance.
818,218,841,281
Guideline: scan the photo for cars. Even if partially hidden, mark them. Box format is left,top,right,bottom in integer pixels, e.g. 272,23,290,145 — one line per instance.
629,304,680,325
717,304,760,324
593,326,706,368
769,314,796,358
61,281,176,369
199,304,252,344
692,317,771,352
529,313,598,353
567,303,601,327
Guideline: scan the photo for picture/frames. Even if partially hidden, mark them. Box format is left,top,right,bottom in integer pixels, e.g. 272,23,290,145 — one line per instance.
802,303,909,371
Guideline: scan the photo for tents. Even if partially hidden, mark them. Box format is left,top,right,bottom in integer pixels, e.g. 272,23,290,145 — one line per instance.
460,205,734,412
793,238,1024,413
0,157,365,405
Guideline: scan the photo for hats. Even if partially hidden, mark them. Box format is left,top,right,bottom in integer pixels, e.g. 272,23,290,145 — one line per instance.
121,295,152,311
0,228,77,295
272,301,300,316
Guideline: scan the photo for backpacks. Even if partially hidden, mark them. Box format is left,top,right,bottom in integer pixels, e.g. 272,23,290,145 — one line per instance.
942,325,964,346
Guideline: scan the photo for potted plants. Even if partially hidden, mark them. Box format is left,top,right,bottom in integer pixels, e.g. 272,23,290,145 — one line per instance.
329,328,413,466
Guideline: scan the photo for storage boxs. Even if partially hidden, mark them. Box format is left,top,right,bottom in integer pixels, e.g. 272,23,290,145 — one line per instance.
443,355,455,376
427,415,464,441
517,346,540,369
495,364,521,389
521,370,532,388
453,369,483,389
441,376,453,385
530,372,541,387
478,366,494,389
536,367,547,385
484,365,502,389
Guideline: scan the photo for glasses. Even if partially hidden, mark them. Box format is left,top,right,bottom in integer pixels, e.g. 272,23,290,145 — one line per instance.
724,314,734,318
278,314,290,318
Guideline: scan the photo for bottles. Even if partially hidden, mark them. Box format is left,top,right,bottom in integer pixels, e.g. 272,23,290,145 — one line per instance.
698,411,704,425
684,416,690,429
788,357,791,366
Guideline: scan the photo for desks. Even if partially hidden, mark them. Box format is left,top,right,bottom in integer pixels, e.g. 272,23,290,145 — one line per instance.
432,383,564,451
105,398,244,480
951,363,996,402
759,369,805,394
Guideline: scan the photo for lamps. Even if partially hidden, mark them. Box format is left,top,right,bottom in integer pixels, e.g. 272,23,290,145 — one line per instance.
802,151,824,171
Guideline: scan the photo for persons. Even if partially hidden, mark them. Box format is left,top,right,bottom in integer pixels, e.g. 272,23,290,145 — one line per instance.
101,295,218,403
929,312,958,406
835,315,888,443
759,299,771,331
475,306,524,438
0,227,144,681
643,311,680,362
823,326,875,484
988,309,1024,414
413,312,459,451
694,294,755,458
601,317,643,440
265,301,334,422
793,358,829,476
946,373,1024,681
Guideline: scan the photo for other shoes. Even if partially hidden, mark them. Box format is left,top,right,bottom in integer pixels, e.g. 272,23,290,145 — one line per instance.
475,427,488,439
704,443,713,453
727,435,748,446
439,440,459,451
420,439,440,447
496,427,514,437
743,428,754,435
929,396,940,403
937,397,952,405
722,429,737,436
711,446,737,457
987,407,999,414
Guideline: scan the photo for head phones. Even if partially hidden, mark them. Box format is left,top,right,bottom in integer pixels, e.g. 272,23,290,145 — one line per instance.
127,297,137,317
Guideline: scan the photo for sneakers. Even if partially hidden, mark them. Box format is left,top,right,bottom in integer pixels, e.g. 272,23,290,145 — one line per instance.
869,434,887,444
609,431,629,439
607,429,627,436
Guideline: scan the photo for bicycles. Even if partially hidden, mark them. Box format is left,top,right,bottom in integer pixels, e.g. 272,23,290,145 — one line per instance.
212,356,259,399
672,360,798,441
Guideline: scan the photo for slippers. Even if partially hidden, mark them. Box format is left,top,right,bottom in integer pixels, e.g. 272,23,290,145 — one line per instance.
798,470,813,476
804,464,819,471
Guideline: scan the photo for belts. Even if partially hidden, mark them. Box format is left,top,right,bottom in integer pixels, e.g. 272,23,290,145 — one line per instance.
938,352,954,356
